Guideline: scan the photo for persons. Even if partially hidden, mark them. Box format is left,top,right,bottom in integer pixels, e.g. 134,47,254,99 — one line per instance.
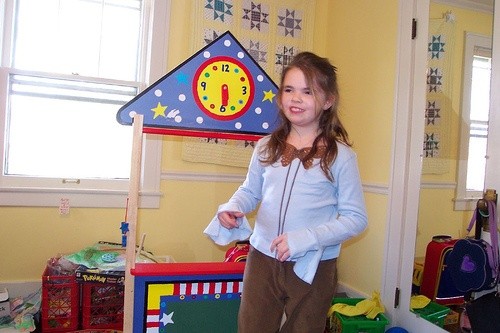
202,51,368,333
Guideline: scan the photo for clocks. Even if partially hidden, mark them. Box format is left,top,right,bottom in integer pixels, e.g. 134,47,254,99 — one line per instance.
192,55,256,120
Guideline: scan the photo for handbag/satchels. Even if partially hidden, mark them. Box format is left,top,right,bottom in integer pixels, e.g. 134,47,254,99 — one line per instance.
448,199,499,293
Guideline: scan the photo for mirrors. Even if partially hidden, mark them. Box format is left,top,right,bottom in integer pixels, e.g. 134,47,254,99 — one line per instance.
406,0,496,333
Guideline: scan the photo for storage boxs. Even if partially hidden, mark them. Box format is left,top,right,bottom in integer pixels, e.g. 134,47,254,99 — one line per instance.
79,273,125,332
41,257,82,332
